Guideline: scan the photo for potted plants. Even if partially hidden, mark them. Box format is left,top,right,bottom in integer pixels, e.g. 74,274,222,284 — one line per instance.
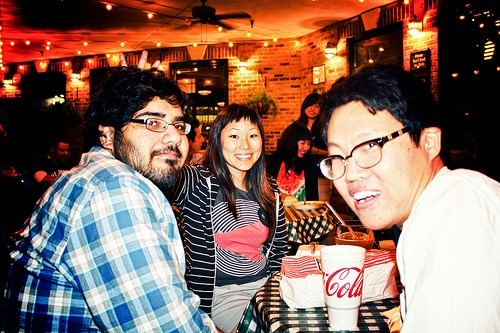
244,92,278,117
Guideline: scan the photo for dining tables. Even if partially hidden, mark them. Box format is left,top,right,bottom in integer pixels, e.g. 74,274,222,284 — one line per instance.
284,197,334,244
236,253,404,333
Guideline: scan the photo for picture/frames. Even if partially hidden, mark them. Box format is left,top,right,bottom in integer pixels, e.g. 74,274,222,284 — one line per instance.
312,64,326,85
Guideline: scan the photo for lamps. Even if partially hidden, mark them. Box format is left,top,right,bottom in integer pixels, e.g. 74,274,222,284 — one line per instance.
324,42,337,59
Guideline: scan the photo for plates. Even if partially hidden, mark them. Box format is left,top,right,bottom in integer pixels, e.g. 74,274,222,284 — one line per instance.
298,201,325,204
293,203,321,210
283,201,299,207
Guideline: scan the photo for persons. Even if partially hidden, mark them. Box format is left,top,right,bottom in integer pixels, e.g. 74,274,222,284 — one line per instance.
316,62,500,333
0,65,220,333
293,92,328,201
184,119,206,165
266,123,311,200
118,48,288,333
35,141,73,183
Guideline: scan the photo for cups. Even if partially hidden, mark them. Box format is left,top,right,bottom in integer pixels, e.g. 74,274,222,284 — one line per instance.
336,220,375,250
321,245,366,331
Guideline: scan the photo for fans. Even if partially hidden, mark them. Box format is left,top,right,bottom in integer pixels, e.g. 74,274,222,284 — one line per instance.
161,0,252,30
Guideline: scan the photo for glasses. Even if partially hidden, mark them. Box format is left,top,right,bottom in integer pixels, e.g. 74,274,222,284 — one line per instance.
315,126,410,181
122,118,192,136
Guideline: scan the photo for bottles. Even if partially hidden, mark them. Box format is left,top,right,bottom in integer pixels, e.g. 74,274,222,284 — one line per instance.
196,106,223,123
